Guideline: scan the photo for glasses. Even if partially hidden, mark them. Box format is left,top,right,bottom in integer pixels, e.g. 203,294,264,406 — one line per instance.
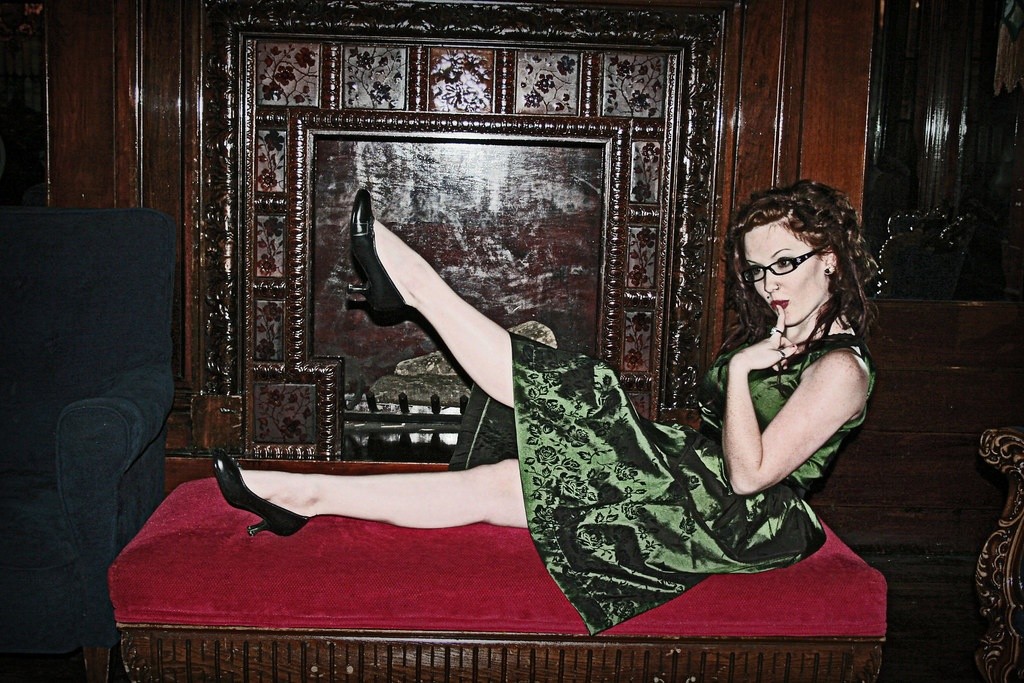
740,244,832,284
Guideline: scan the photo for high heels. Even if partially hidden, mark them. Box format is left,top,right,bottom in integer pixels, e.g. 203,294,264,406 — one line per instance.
213,446,310,537
346,187,407,317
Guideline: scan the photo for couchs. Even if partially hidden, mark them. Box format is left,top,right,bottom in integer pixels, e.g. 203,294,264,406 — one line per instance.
0,204,182,682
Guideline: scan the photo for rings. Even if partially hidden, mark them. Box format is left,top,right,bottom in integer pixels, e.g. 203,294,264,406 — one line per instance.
770,328,783,336
779,350,786,359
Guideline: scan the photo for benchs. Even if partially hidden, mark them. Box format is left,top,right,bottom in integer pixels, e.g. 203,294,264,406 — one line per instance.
106,477,891,683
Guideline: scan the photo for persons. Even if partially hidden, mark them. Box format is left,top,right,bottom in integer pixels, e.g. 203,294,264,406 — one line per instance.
213,180,881,636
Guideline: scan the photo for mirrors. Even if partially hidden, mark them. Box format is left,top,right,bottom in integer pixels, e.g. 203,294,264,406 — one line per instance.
858,0,1024,303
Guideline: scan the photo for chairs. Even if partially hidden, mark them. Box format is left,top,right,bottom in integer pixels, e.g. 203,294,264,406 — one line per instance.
878,206,972,300
